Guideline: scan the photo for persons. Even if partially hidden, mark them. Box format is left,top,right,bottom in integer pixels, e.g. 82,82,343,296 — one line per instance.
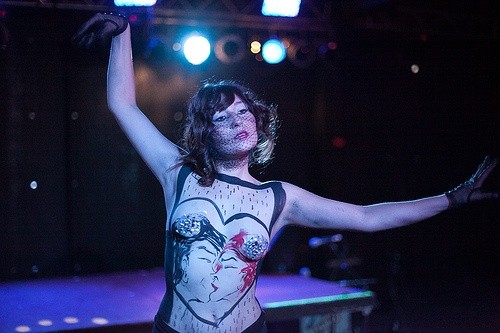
72,11,500,333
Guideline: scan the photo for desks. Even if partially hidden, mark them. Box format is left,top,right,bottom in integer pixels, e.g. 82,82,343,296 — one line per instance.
0,267,375,333
